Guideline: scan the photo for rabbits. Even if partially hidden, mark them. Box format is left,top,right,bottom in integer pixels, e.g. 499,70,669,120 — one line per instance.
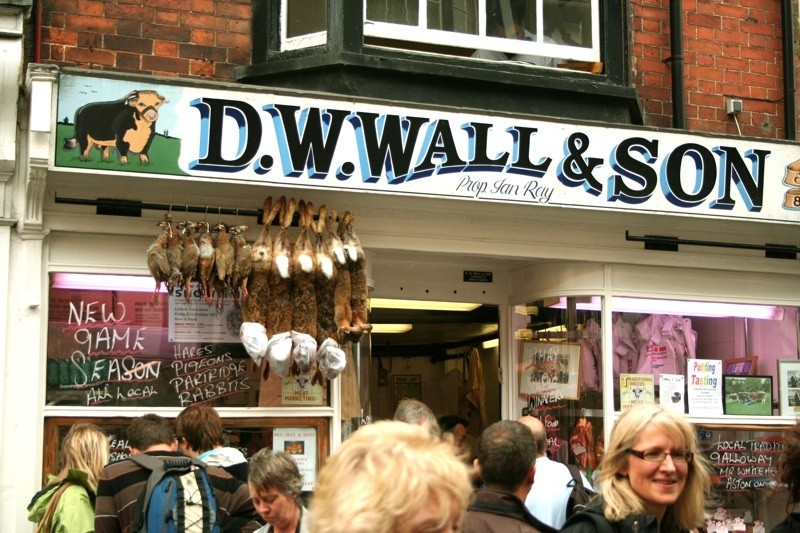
145,195,373,390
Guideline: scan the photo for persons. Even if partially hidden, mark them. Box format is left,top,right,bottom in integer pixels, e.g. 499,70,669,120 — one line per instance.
771,423,800,533
28,421,110,533
248,446,312,533
461,420,543,533
96,413,261,533
174,403,248,482
312,419,475,533
470,0,568,69
395,399,469,451
562,403,713,533
519,416,597,530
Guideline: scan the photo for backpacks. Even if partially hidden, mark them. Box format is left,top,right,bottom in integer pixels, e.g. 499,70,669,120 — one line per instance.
128,455,220,533
565,464,599,521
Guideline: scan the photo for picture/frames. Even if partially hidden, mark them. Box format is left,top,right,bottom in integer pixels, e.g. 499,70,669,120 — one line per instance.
518,338,583,402
778,357,800,416
723,354,759,374
722,374,773,415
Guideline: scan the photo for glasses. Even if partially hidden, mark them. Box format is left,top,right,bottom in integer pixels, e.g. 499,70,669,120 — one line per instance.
623,447,694,462
